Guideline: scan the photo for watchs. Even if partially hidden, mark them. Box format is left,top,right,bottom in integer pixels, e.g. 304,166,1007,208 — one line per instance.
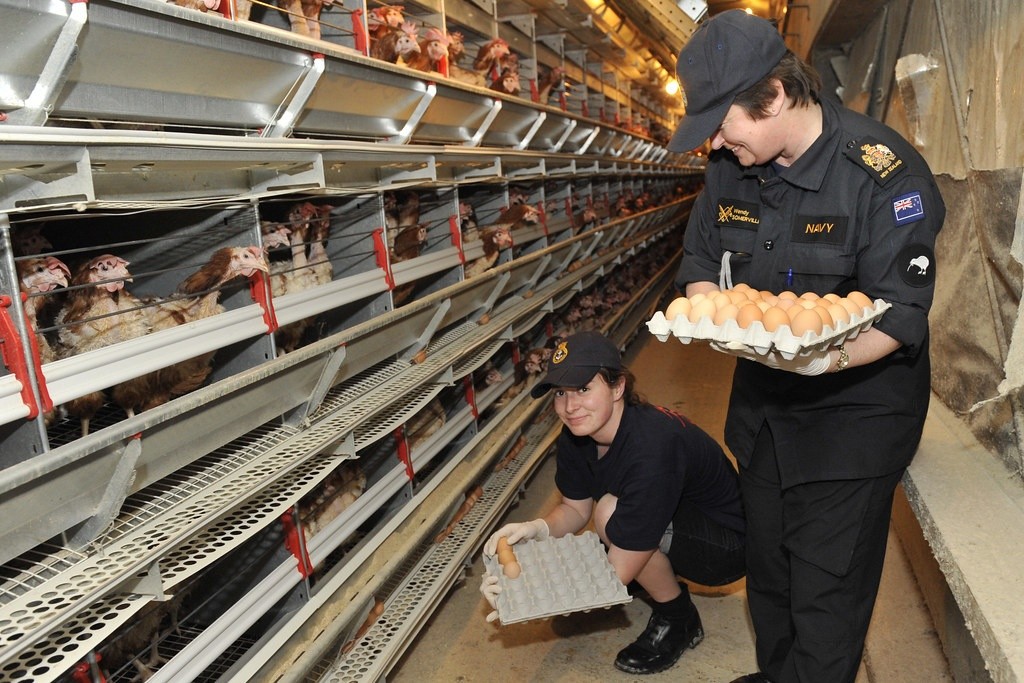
834,343,848,374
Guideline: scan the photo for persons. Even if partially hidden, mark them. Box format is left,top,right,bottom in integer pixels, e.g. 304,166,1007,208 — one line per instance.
482,331,746,675
666,10,947,682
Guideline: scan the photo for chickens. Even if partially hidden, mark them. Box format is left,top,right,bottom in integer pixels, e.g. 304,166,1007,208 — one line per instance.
0,180,706,683
168,1,706,157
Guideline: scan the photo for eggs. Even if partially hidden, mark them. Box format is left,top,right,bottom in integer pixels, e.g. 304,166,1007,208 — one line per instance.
664,282,873,338
352,246,617,641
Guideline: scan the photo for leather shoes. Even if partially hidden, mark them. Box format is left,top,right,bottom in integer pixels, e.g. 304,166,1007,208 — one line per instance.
614,581,704,676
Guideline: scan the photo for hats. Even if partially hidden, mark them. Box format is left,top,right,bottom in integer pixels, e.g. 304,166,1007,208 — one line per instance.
531,330,622,398
665,8,788,152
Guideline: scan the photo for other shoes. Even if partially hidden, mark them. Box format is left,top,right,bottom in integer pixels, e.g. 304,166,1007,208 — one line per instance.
730,672,770,683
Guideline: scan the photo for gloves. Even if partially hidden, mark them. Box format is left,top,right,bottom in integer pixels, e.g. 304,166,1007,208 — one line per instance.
483,518,550,560
709,343,831,376
480,571,502,623
